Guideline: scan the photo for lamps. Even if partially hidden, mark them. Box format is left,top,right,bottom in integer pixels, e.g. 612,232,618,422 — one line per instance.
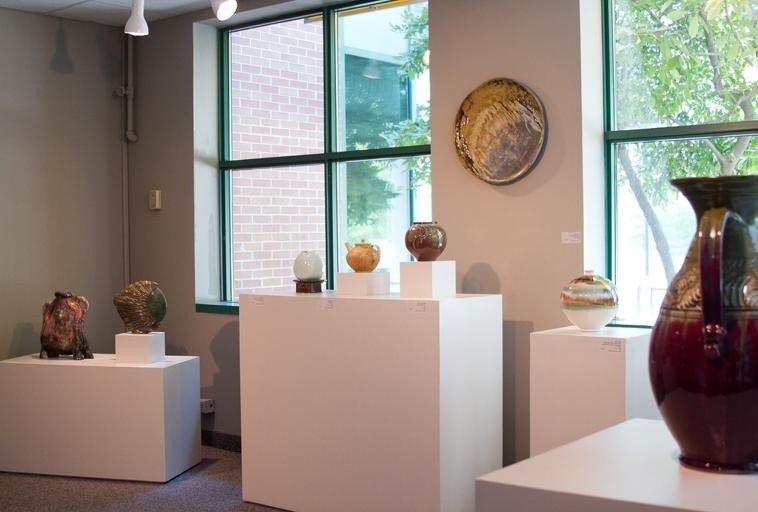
208,0,238,22
362,59,386,82
124,0,150,38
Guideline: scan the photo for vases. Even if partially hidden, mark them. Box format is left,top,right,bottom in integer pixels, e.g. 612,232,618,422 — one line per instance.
559,269,622,333
647,174,757,475
405,221,448,261
293,251,323,280
